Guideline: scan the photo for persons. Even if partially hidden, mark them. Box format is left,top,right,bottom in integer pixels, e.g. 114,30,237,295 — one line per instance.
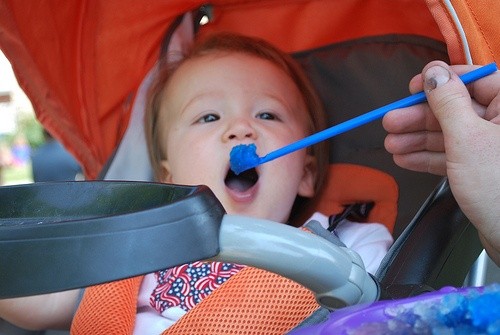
0,32,398,334
1,89,21,154
29,126,89,183
382,58,499,270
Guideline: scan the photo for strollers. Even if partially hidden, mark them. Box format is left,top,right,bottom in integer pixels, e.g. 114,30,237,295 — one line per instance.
2,1,500,335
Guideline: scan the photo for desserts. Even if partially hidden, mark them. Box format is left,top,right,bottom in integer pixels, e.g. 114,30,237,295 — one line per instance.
229,143,260,176
